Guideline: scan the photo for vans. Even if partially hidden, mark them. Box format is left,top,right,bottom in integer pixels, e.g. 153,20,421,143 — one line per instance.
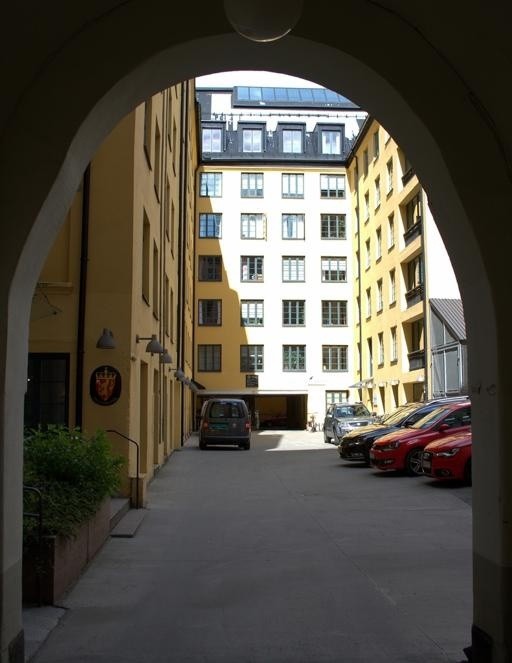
195,398,252,450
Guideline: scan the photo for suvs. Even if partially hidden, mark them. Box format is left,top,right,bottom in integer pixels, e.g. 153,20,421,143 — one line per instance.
321,401,375,445
337,395,469,461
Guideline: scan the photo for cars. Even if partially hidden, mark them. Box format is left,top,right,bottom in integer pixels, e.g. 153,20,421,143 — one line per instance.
421,432,472,484
369,399,471,476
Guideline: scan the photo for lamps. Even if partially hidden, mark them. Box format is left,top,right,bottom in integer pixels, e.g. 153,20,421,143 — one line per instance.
136,333,185,383
222,1,304,44
95,327,117,351
